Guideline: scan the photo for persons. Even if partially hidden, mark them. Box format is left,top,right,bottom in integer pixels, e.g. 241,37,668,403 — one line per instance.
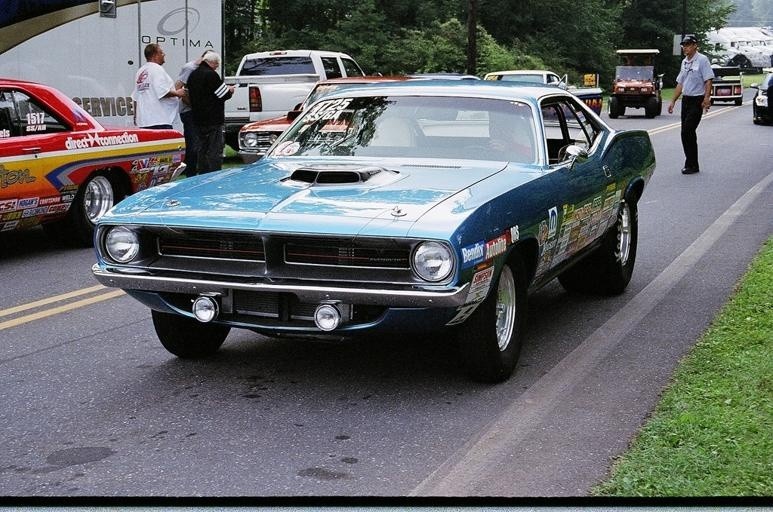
668,34,715,177
177,50,216,130
184,52,235,178
131,44,186,134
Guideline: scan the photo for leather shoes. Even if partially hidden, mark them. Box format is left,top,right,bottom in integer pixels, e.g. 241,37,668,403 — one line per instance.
682,167,699,174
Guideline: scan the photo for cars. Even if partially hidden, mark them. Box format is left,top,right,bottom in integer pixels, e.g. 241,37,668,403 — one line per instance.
92,80,656,385
0,77,187,245
750,74,773,125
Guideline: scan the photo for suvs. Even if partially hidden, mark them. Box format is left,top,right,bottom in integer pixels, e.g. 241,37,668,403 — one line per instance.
238,76,415,163
607,48,662,118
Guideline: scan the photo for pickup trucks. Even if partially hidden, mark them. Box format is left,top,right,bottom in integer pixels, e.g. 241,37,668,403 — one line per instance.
483,71,604,120
224,49,366,151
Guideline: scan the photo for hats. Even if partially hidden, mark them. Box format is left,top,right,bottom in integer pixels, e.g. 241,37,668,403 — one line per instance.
679,36,697,44
